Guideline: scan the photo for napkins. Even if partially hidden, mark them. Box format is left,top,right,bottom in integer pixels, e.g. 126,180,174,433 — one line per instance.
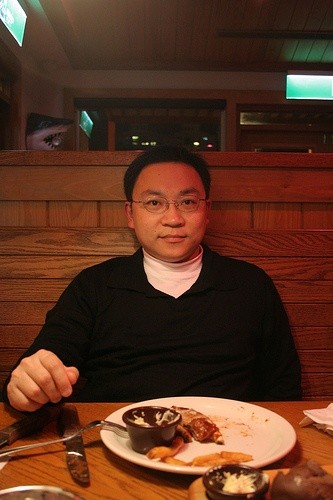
301,402,333,436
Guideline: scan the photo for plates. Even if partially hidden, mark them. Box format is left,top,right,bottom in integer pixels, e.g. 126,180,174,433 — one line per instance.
188,464,333,500
98,395,298,475
302,407,333,425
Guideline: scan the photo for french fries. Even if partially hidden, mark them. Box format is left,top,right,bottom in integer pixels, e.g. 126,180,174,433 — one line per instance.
147,438,253,467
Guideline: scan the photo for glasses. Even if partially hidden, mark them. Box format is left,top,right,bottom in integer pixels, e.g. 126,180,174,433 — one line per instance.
130,195,208,213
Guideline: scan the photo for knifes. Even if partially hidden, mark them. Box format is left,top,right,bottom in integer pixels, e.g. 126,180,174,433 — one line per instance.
58,405,90,487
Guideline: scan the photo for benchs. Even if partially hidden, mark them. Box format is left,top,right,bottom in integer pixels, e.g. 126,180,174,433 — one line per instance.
0,227,333,402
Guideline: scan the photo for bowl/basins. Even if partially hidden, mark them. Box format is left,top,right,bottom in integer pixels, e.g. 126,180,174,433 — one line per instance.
202,462,269,500
120,406,181,453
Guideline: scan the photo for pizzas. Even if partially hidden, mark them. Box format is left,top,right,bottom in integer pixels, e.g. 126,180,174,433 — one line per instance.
171,406,224,444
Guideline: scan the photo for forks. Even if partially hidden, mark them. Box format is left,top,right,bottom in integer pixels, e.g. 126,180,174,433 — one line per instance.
1,419,130,459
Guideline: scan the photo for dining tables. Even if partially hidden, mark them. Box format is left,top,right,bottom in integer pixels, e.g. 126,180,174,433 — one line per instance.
0,402,333,500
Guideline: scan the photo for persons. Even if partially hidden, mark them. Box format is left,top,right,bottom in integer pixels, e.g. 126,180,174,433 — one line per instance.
0,145,303,413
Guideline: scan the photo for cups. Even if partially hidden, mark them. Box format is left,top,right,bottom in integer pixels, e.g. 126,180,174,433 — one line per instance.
1,485,81,500
